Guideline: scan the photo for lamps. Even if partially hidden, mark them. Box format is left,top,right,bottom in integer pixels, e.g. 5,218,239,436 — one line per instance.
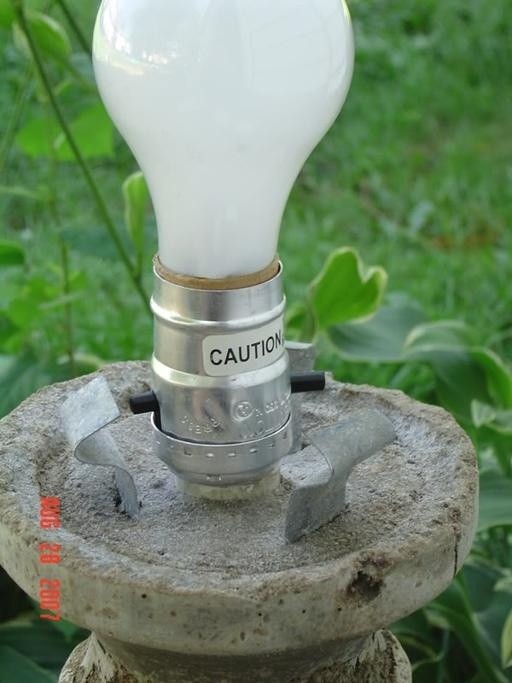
87,0,354,497
